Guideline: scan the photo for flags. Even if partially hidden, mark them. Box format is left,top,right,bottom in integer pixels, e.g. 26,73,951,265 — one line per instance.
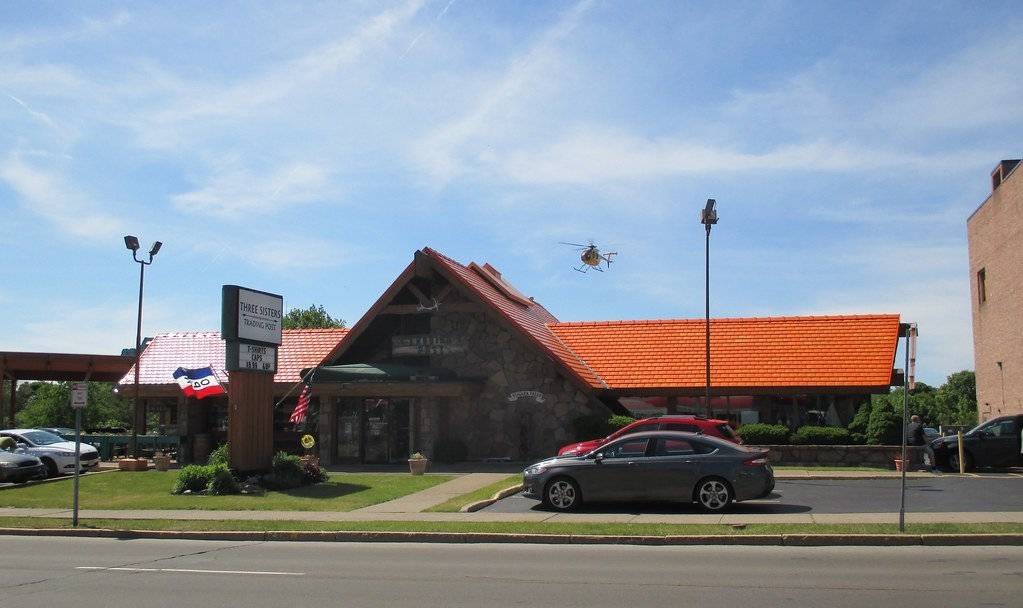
173,364,225,398
288,373,316,426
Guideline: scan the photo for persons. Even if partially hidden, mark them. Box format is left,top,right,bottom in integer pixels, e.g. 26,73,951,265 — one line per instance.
906,416,943,476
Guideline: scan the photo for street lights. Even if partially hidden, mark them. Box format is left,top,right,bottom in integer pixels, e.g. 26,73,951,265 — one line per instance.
124,236,157,459
698,198,719,418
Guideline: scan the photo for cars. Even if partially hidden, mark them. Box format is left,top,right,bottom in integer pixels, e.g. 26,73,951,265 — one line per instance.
930,413,1023,472
522,431,777,512
0,448,46,484
0,427,100,476
556,413,746,458
922,427,937,434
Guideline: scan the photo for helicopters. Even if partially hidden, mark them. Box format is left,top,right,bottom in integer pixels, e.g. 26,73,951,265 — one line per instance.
557,238,618,274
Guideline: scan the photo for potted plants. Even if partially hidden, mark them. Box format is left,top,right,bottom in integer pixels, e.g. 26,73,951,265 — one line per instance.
153,450,172,471
408,452,428,476
300,454,320,468
894,453,910,472
